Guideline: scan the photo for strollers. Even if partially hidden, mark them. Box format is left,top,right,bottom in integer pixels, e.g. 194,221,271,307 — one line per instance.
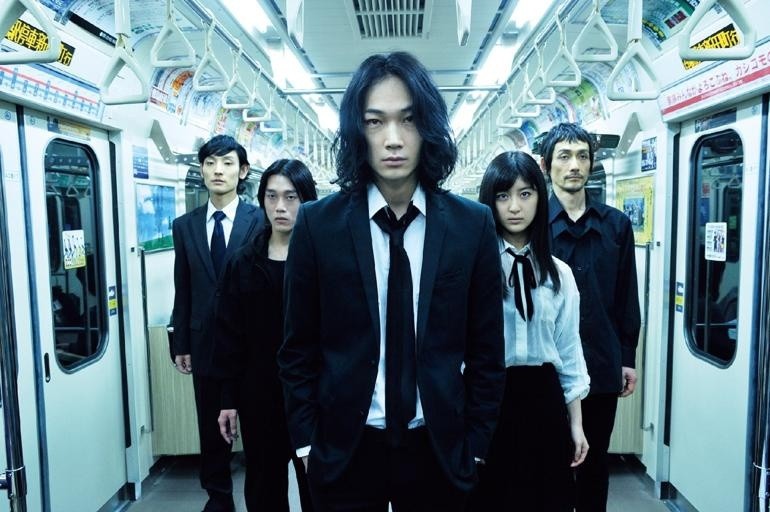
504,247,538,321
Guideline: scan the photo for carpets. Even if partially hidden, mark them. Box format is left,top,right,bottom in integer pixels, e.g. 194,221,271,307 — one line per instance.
370,199,421,448
210,209,227,284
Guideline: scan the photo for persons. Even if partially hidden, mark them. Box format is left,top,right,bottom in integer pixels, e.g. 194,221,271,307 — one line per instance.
477,149,592,511
217,158,319,511
539,122,641,512
276,47,509,512
165,134,270,511
